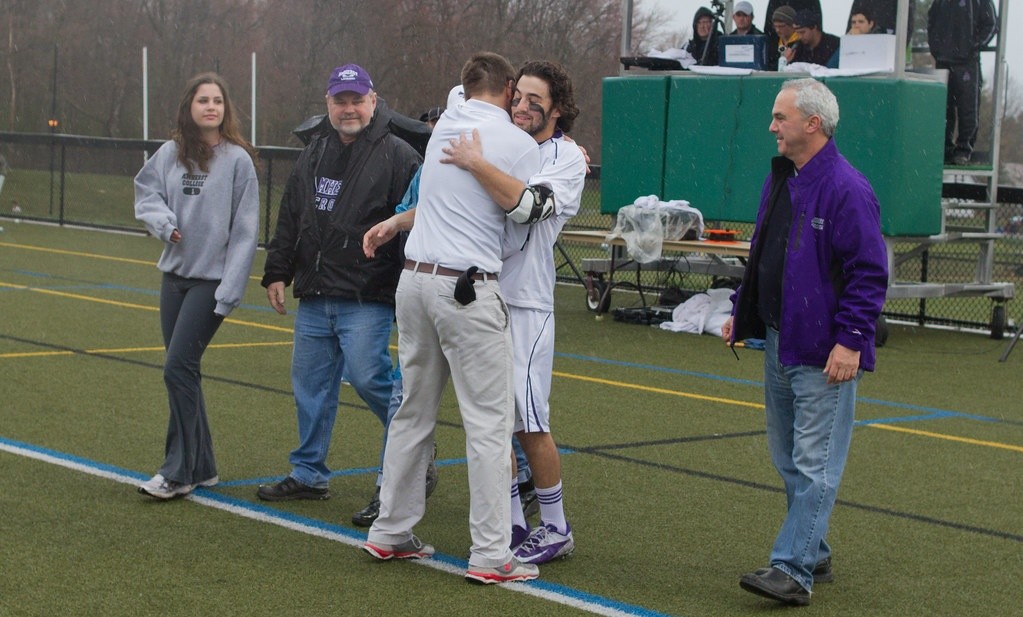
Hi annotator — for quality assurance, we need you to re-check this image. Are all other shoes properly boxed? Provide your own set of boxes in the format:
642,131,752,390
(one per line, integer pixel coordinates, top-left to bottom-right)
945,151,954,165
954,148,975,165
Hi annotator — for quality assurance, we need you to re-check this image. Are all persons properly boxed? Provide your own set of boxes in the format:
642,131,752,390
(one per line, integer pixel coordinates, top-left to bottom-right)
729,2,762,35
351,85,539,527
134,72,263,499
362,51,592,585
721,78,889,606
363,61,587,566
767,5,797,71
825,9,887,68
783,8,840,66
926,0,999,164
686,7,724,66
257,64,439,500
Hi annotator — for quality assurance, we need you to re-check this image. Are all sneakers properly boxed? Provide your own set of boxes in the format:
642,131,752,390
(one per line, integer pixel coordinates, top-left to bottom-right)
256,475,331,500
513,522,574,563
363,539,436,561
191,472,219,489
352,486,382,527
424,442,441,499
466,553,540,585
519,479,540,517
140,473,198,498
509,524,533,550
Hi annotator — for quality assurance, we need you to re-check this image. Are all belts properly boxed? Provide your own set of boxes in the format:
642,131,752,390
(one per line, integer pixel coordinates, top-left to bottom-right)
403,259,498,280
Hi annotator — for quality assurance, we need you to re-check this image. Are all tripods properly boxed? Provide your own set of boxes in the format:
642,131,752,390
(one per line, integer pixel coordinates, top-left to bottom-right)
699,0,728,66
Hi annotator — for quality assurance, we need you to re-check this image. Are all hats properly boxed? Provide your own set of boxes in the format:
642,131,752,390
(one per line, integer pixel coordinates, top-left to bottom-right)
791,8,820,31
731,1,753,17
326,63,373,98
772,6,797,26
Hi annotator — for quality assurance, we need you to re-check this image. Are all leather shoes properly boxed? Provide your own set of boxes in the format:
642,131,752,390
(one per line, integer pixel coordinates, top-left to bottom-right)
741,564,811,606
756,558,833,584
